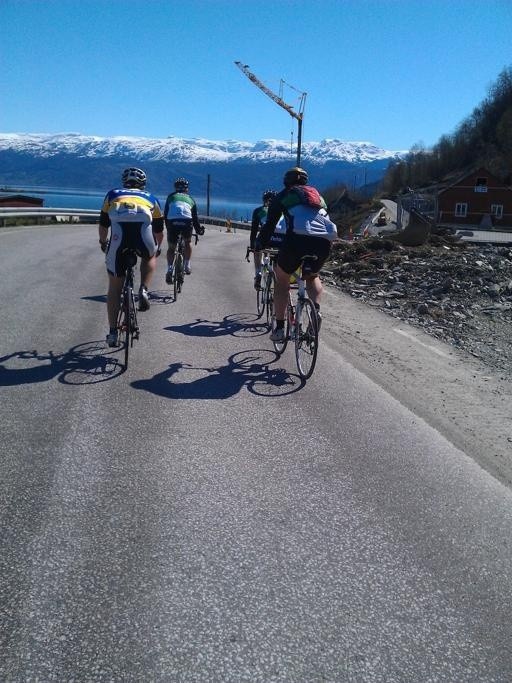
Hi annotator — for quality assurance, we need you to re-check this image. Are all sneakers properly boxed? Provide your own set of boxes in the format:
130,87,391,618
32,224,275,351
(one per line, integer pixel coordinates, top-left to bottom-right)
269,327,285,341
105,332,118,348
306,309,323,337
138,284,151,311
253,271,262,290
165,271,174,285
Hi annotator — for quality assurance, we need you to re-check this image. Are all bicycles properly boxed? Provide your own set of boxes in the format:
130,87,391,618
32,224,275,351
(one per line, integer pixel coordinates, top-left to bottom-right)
167,227,204,301
244,245,319,380
104,252,147,370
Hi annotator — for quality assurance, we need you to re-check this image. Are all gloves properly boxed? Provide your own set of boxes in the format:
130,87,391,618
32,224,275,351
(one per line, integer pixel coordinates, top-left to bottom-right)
98,239,111,254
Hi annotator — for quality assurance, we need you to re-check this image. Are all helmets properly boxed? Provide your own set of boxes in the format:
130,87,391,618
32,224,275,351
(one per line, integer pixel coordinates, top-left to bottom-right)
282,166,308,186
121,166,147,191
262,189,281,206
174,176,189,193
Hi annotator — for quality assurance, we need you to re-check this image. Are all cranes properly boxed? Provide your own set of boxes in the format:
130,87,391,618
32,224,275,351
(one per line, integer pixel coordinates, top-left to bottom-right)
233,60,309,167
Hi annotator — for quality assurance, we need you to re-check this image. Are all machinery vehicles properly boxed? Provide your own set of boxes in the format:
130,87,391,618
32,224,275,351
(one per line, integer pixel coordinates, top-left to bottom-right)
378,212,387,225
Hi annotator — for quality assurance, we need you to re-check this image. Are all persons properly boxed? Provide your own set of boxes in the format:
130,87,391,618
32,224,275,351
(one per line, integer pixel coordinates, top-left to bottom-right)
249,188,287,290
255,165,335,342
162,177,206,284
98,164,164,346
